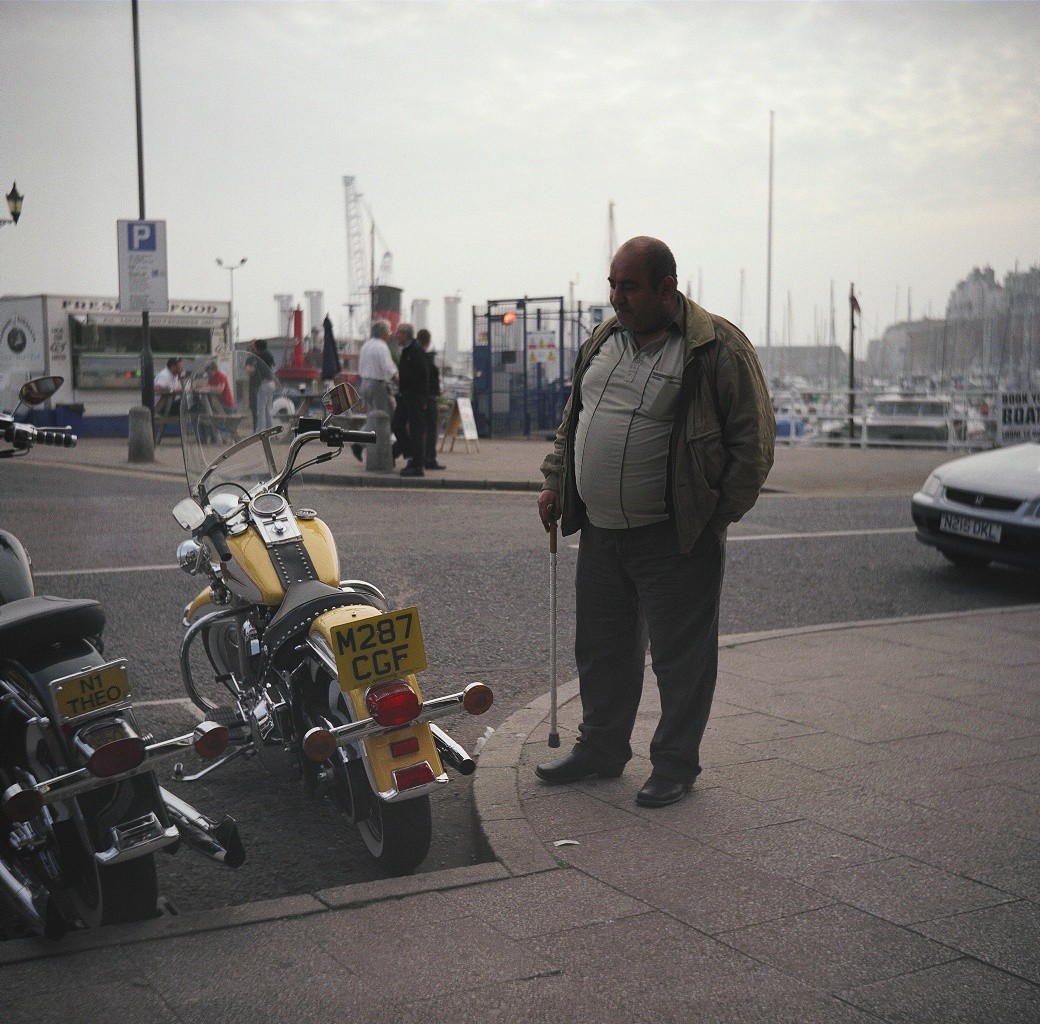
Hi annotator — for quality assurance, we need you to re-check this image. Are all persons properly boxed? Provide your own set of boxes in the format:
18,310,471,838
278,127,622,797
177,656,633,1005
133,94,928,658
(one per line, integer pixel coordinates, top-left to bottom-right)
351,319,447,476
246,338,272,434
538,236,776,805
772,407,818,436
152,355,232,417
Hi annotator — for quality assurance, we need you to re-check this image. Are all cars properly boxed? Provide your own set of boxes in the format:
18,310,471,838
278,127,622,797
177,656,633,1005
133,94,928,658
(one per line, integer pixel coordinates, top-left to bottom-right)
910,436,1040,574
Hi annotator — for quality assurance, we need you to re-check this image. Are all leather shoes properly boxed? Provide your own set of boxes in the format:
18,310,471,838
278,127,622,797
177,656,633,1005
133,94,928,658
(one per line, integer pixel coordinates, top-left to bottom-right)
635,772,696,805
535,748,627,782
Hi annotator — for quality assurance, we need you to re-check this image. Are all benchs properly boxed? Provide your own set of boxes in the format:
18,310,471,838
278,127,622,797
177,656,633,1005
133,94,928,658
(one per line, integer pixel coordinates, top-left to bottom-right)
152,411,243,428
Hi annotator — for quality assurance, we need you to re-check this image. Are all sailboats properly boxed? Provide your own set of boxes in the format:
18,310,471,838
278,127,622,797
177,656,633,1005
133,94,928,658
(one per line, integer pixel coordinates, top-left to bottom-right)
722,110,1035,448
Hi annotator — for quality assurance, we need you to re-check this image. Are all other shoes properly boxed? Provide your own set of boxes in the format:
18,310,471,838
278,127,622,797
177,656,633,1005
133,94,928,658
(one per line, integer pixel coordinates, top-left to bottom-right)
426,461,447,471
399,466,426,478
351,444,363,462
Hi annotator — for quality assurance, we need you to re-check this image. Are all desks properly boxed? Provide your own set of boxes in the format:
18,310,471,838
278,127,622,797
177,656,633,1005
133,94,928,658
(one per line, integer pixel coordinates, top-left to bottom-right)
155,383,224,446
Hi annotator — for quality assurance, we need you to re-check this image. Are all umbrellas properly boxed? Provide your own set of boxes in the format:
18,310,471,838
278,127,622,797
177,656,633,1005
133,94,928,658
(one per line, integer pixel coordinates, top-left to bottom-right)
321,318,341,391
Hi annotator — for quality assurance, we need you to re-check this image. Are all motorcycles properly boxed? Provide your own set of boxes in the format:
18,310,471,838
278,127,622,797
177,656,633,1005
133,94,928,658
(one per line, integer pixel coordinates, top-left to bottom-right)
0,376,252,943
165,381,495,877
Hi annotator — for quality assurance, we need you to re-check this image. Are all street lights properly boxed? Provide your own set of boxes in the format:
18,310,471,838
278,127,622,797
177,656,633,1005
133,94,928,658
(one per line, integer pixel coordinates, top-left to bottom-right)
215,257,250,380
